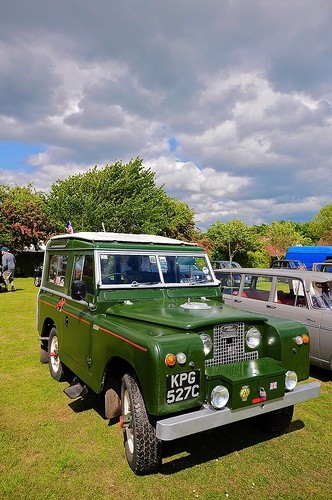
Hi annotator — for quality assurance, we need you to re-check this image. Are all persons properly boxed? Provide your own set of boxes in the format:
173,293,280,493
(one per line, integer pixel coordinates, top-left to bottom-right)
0,247,16,290
292,265,315,298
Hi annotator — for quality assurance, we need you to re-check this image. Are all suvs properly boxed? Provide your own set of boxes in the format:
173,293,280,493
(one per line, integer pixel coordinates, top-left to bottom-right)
36,232,321,477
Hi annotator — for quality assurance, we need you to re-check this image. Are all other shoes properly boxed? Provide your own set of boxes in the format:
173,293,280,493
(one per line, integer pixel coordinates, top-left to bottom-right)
11,286,14,291
3,288,8,292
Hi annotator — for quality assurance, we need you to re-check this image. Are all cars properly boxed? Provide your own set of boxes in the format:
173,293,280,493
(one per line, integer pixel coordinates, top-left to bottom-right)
262,259,302,282
209,260,244,288
34,256,71,288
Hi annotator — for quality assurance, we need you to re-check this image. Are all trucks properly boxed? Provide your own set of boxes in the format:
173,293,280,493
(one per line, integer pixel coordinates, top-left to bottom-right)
286,245,332,274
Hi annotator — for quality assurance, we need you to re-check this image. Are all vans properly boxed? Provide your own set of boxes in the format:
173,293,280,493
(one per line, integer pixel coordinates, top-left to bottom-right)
209,268,332,375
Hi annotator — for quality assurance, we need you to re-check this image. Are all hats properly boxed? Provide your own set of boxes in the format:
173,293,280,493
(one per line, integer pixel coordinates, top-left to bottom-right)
0,247,7,251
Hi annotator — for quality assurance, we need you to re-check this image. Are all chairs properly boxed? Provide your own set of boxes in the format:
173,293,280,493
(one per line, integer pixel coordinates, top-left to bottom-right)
241,289,259,299
123,269,143,283
276,290,295,304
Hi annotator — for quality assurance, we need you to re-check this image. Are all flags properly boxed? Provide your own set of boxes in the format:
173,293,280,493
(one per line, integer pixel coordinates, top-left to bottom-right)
65,221,74,234
